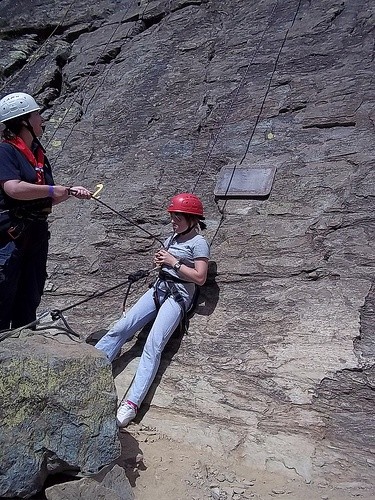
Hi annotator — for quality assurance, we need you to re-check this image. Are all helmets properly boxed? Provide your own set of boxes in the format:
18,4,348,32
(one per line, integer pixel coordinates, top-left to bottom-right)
168,194,203,215
0,92,41,123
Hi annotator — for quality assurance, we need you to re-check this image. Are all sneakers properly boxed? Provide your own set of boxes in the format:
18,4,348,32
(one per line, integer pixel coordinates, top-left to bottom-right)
116,402,136,428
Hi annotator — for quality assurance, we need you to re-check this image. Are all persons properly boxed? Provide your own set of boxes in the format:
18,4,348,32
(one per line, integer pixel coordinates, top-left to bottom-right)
94,192,210,428
0,92,94,332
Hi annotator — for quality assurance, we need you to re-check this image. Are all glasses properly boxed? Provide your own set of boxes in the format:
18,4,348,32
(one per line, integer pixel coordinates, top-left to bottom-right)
170,214,185,221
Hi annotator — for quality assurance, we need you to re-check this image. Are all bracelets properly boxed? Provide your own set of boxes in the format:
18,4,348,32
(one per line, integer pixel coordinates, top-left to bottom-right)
173,261,183,272
48,185,54,198
